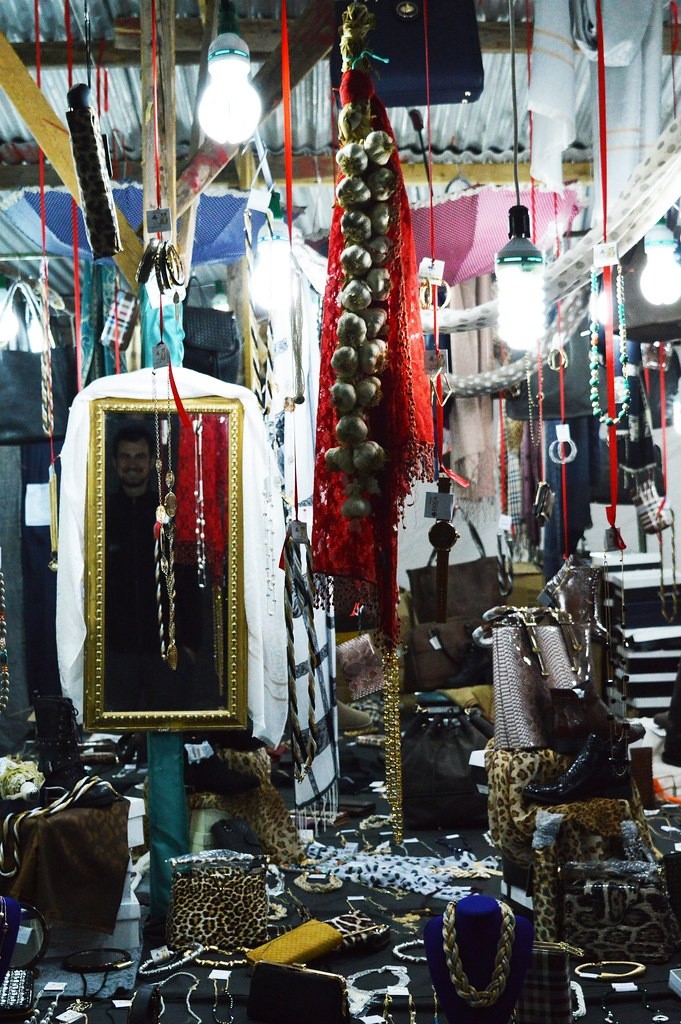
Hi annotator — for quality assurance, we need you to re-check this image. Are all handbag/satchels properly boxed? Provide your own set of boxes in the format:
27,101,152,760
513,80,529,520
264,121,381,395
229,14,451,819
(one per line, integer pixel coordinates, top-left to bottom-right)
0,968,34,1021
164,817,269,950
247,227,681,1024
127,984,160,1024
328,0,484,109
0,895,21,981
178,277,239,383
0,280,68,446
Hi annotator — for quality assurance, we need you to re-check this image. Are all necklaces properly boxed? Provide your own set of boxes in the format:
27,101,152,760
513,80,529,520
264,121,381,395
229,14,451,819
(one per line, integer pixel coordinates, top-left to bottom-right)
279,532,322,779
193,412,210,592
149,339,187,679
499,310,677,632
0,775,673,1024
442,897,517,1009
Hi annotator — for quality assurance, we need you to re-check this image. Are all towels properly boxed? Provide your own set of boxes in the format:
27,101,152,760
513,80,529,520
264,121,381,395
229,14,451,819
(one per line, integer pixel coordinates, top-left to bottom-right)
1,796,132,937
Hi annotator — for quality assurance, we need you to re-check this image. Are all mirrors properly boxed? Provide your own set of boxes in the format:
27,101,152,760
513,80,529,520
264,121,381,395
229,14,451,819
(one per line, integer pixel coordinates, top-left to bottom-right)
84,399,247,730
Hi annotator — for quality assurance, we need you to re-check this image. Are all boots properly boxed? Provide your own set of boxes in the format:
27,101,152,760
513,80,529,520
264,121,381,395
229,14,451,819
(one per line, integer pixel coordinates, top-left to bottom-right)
32,694,115,805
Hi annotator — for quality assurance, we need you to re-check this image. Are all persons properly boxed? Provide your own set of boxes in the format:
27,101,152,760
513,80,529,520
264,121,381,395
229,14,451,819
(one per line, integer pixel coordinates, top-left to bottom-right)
106,424,178,711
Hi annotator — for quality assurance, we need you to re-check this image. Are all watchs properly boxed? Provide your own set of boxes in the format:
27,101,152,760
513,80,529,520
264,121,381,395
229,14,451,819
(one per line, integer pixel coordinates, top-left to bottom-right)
427,434,461,627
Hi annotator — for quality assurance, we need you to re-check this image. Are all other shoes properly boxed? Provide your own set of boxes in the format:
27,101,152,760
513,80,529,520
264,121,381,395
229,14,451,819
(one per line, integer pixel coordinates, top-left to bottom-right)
183,741,261,794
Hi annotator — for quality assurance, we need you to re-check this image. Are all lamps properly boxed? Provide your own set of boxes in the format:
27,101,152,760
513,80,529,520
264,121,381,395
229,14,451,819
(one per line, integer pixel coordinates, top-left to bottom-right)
196,1,264,146
493,1,550,352
640,225,681,308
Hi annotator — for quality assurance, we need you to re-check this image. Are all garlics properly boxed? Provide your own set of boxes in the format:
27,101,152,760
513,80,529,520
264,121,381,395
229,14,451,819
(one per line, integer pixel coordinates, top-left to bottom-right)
324,131,395,520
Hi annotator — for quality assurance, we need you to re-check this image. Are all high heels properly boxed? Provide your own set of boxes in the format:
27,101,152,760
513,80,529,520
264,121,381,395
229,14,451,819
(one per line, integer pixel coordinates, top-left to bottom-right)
88,731,147,780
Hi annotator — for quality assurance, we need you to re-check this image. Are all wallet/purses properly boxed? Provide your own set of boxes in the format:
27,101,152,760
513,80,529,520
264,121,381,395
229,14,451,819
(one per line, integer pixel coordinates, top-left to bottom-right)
99,265,139,350
